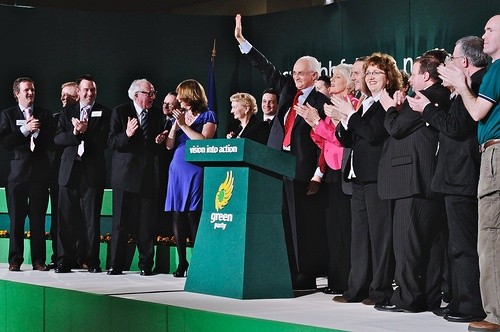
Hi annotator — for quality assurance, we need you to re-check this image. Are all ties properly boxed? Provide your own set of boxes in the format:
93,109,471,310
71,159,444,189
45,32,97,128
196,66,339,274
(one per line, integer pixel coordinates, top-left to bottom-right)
139,109,147,133
282,89,303,147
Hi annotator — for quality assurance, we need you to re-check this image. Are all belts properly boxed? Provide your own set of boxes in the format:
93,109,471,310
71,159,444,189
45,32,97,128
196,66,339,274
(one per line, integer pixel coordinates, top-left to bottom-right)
478,138,500,152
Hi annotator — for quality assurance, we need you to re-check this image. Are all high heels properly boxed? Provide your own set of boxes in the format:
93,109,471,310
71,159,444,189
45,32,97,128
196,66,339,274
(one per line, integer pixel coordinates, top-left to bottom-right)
172,261,190,278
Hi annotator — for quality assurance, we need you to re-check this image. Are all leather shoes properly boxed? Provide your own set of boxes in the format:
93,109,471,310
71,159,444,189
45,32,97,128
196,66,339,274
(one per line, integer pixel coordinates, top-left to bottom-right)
373,302,420,312
466,319,500,331
432,305,484,322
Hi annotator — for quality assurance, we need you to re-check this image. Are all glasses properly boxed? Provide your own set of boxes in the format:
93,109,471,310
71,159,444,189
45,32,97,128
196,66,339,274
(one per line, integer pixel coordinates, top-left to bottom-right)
138,89,158,98
451,55,466,63
364,69,386,77
162,100,175,107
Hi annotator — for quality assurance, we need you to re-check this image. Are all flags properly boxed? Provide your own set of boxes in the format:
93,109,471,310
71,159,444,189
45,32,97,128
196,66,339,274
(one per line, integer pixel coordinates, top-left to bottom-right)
207,58,215,138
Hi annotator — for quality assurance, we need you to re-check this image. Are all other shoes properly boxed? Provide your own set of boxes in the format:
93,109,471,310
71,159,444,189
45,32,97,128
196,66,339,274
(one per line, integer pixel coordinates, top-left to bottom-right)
140,267,151,276
364,295,384,304
9,264,21,271
332,294,368,302
55,265,71,273
33,263,50,272
87,263,102,274
320,282,346,292
107,267,122,275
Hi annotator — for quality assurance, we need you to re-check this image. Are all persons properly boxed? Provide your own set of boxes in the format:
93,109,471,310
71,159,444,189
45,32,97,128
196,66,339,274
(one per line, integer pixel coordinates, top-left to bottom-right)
162,79,218,277
234,14,333,291
225,92,267,143
294,14,500,332
261,90,278,125
54,77,112,273
0,78,57,271
151,90,184,274
48,82,88,268
108,79,167,276
